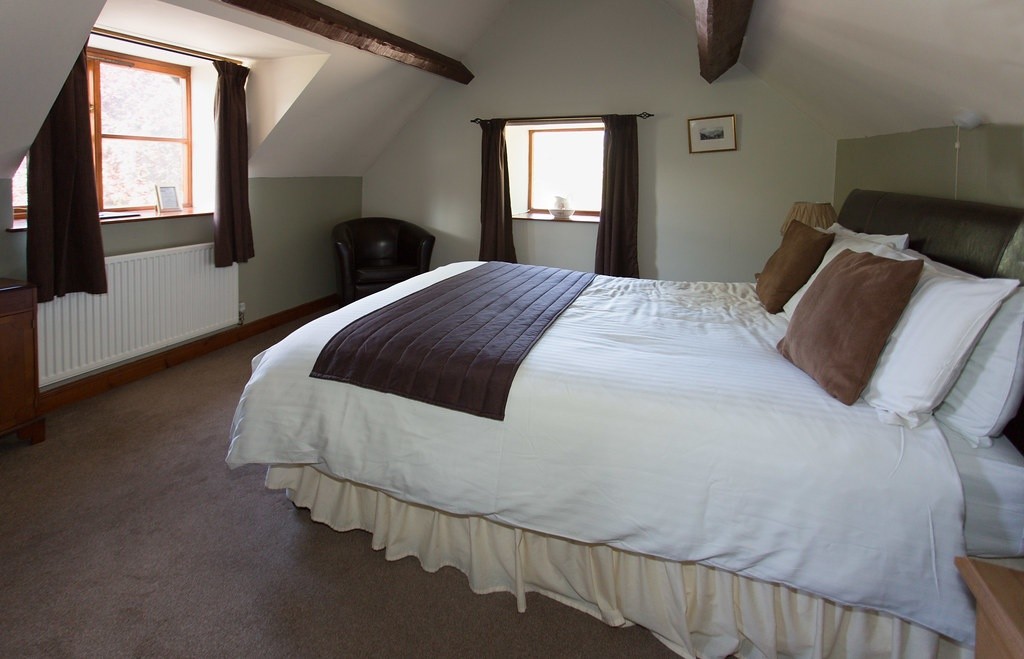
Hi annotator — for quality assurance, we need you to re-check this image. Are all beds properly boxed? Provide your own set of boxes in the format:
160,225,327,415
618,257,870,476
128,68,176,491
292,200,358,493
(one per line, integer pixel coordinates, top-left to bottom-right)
224,123,1024,659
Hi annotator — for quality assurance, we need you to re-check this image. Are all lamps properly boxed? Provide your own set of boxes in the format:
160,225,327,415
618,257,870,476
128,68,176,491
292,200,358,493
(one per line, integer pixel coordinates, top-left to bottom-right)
779,202,838,233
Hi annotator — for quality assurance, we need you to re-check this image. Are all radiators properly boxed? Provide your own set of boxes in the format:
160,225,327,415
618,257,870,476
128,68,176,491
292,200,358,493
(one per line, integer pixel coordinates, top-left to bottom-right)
38,243,240,394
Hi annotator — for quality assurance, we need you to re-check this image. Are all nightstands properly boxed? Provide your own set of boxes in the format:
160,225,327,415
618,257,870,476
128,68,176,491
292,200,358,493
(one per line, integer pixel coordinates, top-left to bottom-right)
953,557,1024,659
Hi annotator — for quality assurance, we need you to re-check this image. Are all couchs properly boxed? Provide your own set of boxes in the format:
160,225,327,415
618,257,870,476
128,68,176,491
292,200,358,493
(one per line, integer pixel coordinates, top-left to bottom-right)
332,217,436,308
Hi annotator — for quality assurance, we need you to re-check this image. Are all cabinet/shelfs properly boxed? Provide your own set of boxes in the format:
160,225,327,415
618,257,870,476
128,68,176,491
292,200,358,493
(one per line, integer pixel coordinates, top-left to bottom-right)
0,278,45,447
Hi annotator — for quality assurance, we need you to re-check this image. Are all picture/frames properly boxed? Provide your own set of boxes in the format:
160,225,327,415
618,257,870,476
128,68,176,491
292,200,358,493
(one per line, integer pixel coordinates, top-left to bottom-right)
687,113,737,154
155,185,182,213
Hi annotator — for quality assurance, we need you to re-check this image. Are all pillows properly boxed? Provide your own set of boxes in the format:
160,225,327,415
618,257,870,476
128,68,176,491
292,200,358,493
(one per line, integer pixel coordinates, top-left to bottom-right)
860,245,1020,429
826,223,910,259
782,227,896,318
776,249,925,407
905,250,1024,447
756,219,834,314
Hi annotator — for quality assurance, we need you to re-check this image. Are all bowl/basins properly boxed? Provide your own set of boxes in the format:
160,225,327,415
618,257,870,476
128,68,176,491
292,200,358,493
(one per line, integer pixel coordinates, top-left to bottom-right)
549,210,575,219
